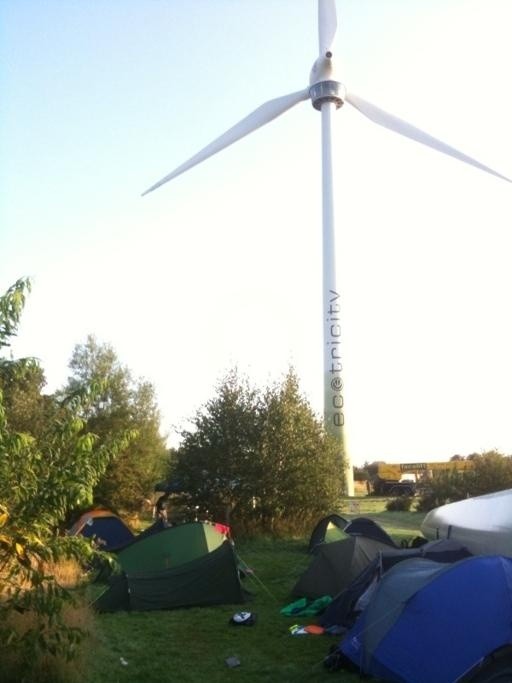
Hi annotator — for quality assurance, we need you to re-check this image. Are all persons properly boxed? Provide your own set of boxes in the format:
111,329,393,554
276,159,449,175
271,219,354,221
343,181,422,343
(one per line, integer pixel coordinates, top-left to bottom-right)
155,491,172,526
